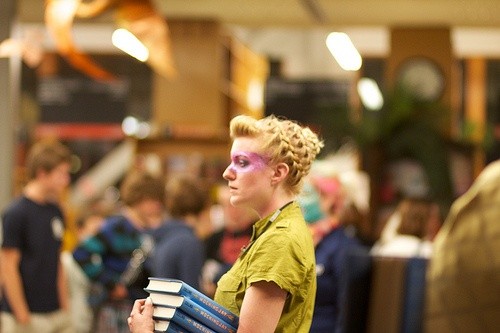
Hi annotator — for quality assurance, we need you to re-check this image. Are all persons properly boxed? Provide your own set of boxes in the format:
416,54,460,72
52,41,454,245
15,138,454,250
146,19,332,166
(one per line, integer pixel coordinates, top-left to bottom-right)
128,115,323,333
73,177,166,332
296,160,371,333
150,176,210,291
1,141,69,333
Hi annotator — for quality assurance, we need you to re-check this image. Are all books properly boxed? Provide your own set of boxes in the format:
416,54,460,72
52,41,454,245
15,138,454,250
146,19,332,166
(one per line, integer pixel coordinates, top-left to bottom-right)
143,278,240,333
